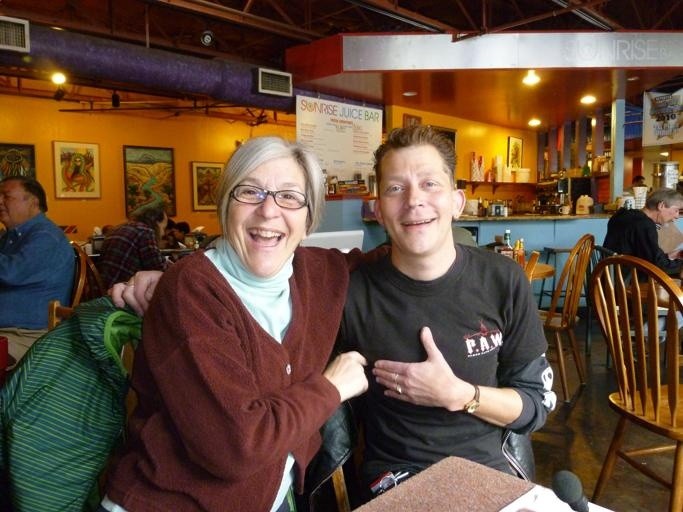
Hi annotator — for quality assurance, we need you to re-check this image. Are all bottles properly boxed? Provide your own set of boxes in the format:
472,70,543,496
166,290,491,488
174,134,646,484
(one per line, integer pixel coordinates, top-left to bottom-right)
513,239,519,260
478,197,483,216
504,205,508,217
518,238,526,269
583,159,590,176
498,230,514,260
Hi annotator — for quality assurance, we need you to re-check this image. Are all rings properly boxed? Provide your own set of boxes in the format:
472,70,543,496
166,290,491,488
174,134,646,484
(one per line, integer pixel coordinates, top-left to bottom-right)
396,384,401,394
125,284,134,288
394,374,397,382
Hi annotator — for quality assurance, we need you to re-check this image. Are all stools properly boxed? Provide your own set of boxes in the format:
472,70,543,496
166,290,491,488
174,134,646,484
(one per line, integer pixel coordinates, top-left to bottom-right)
539,246,597,318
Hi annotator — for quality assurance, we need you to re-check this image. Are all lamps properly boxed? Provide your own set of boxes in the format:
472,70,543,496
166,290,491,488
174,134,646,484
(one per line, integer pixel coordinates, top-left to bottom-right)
200,29,214,45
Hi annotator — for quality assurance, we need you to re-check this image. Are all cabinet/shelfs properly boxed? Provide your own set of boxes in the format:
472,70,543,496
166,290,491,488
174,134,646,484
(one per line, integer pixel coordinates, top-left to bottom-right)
458,178,555,191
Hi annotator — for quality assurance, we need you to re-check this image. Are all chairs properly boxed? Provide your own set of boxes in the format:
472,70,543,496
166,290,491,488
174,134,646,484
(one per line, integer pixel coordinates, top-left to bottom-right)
585,244,636,368
537,234,595,403
67,241,86,308
587,253,683,512
87,256,106,300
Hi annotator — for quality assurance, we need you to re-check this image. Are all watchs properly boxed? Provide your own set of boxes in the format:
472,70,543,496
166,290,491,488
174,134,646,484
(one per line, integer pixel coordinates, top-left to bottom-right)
465,384,480,415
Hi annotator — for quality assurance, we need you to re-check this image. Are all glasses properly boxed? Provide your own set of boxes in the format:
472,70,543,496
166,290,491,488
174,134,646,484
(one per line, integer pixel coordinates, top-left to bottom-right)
230,184,308,210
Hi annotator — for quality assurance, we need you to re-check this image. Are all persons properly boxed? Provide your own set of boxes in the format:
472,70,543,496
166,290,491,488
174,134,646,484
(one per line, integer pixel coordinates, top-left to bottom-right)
678,181,683,194
163,218,190,260
97,137,368,512
102,224,112,234
626,175,645,195
97,206,174,295
109,124,557,506
0,175,79,375
603,188,683,363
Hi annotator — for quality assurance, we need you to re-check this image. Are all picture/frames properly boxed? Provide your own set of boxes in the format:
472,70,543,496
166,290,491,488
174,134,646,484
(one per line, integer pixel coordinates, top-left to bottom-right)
52,140,102,199
0,143,36,180
123,143,177,218
191,161,227,211
507,136,523,168
